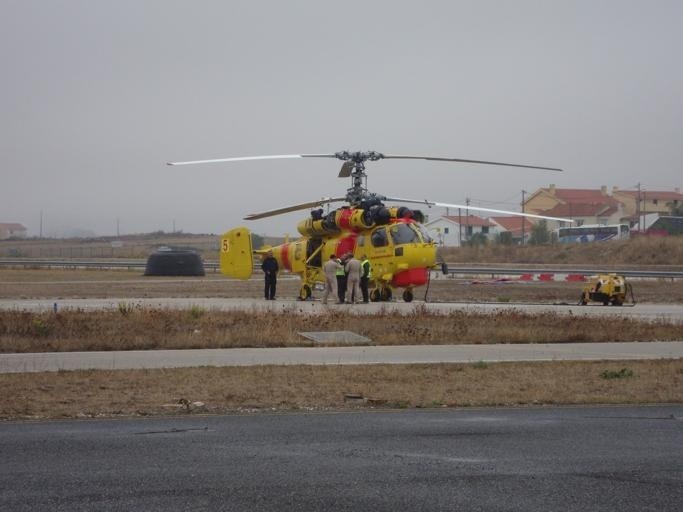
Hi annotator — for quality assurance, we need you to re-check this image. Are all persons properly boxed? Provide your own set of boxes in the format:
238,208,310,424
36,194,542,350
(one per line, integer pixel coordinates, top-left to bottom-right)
336,252,347,302
358,253,372,303
260,251,279,300
343,253,361,304
320,253,342,305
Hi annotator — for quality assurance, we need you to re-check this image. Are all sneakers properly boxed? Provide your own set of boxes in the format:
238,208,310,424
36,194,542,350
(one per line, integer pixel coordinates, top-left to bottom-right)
335,298,368,304
265,297,275,300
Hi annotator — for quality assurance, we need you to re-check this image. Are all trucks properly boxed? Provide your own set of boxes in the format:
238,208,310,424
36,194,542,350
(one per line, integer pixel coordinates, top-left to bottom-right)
582,274,627,305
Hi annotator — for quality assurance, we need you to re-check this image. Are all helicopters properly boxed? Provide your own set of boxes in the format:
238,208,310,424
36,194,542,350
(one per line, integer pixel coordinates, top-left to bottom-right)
166,153,575,302
